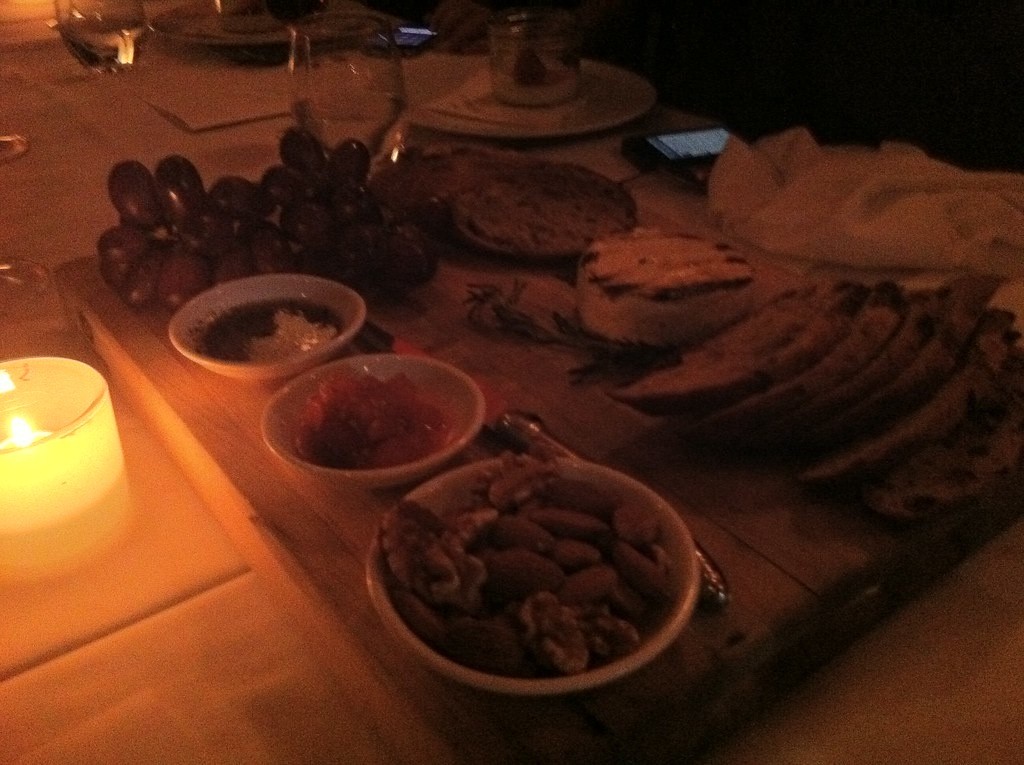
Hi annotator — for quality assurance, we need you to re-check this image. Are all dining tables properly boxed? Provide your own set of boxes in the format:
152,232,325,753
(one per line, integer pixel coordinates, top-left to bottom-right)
0,0,1023,765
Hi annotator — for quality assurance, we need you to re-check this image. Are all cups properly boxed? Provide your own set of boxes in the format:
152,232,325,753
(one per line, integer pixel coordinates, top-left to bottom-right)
286,12,406,156
487,13,578,108
53,0,146,73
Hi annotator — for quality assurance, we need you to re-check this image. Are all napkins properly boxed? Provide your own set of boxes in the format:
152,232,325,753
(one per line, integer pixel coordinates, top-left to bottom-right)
426,64,603,125
711,126,1024,278
134,60,292,132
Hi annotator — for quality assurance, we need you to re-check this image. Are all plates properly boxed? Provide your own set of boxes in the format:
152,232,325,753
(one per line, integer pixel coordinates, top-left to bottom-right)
146,0,293,45
403,53,657,139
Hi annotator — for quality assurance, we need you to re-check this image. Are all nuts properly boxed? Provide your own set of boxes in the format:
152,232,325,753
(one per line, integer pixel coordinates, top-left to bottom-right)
379,454,677,677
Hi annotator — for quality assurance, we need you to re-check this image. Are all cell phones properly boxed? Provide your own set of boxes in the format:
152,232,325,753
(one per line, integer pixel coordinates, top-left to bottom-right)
624,126,730,173
363,26,440,59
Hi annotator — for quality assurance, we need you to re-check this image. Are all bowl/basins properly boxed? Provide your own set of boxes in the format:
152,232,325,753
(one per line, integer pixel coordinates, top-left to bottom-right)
365,455,702,697
168,272,369,383
261,353,486,489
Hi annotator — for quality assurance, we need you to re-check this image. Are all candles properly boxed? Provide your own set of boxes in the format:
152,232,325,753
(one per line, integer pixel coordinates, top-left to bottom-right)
0,357,134,574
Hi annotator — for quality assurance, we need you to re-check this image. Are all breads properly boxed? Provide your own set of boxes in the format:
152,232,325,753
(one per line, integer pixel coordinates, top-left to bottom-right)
605,269,1024,523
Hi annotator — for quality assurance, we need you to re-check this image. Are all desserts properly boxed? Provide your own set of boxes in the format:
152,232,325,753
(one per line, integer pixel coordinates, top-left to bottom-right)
576,225,751,348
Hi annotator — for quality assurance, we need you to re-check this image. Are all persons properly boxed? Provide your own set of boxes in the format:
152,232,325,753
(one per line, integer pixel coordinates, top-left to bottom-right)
262,0,635,45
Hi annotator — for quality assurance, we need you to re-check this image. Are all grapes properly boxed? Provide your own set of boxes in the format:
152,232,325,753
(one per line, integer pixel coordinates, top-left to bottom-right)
98,127,438,314
